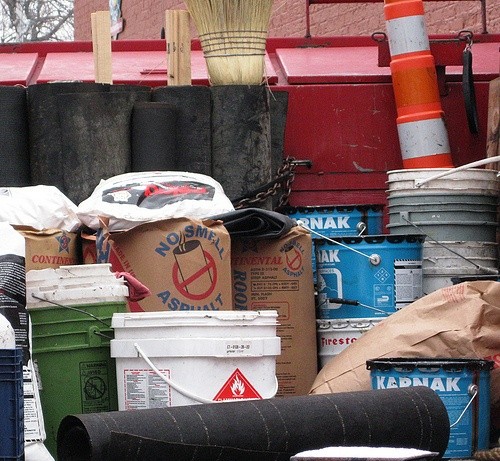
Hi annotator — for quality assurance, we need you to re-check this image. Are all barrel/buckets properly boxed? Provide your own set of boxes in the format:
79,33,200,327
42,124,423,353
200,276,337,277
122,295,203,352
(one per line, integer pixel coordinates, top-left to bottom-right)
282,203,386,287
26,263,281,460
317,297,390,371
385,156,499,297
311,233,427,321
366,357,494,461
